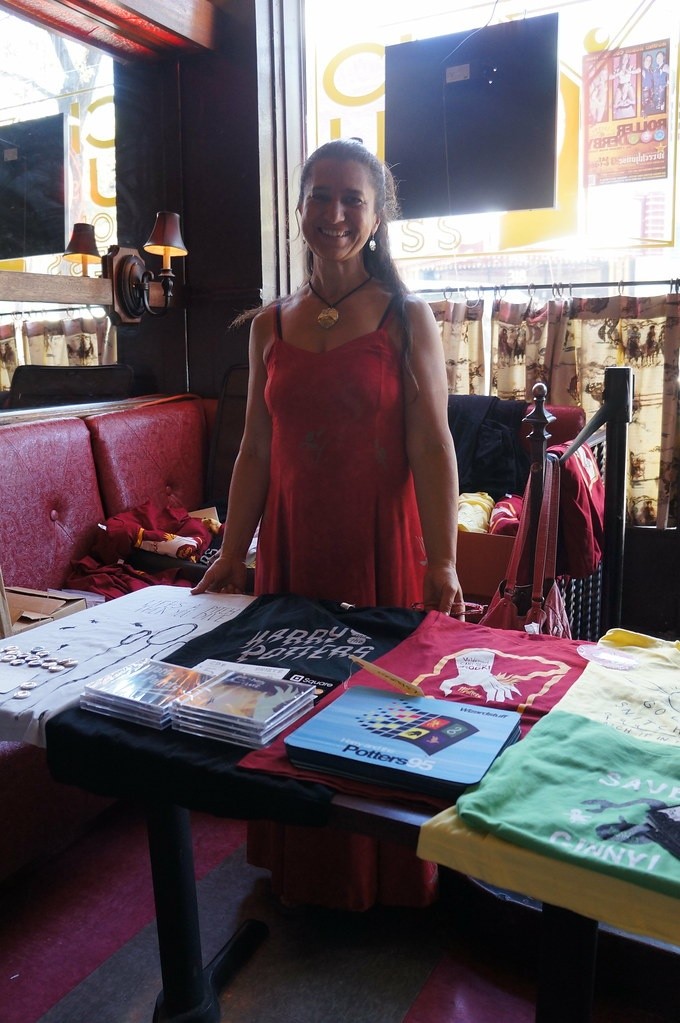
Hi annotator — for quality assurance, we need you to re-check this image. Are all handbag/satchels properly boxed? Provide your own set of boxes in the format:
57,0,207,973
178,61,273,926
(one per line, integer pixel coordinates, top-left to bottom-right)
477,452,574,639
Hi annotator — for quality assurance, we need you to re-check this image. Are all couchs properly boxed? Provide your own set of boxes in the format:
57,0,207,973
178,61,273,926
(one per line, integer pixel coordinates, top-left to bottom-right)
0,397,588,858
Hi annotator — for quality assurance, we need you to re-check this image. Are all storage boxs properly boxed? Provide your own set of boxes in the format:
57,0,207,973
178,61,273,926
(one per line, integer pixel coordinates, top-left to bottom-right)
456,531,516,600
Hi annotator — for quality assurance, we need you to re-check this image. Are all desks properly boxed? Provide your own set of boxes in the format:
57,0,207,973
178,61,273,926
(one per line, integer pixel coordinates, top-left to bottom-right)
0,581,680,1023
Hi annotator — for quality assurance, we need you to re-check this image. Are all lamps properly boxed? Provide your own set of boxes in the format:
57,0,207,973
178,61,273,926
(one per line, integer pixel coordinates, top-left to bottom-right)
61,223,102,277
108,210,190,324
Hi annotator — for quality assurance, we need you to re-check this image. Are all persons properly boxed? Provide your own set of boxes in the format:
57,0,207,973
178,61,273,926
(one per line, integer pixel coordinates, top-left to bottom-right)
188,137,467,934
587,50,670,122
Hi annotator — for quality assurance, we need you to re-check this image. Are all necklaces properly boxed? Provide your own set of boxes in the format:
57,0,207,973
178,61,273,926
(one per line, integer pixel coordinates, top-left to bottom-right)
307,271,373,330
